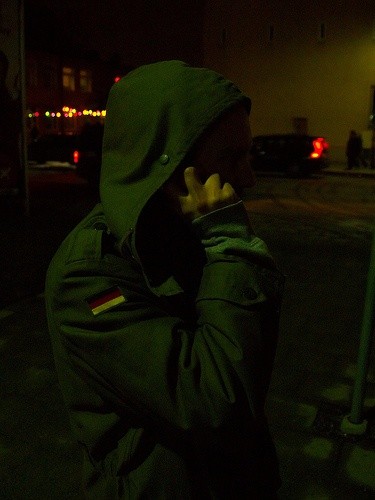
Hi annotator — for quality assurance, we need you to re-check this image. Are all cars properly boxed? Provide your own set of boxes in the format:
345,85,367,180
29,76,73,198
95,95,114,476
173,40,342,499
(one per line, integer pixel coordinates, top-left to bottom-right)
27,133,77,165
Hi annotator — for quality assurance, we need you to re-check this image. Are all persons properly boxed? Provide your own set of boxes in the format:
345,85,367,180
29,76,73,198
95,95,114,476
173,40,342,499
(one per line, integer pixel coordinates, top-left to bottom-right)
38,59,295,500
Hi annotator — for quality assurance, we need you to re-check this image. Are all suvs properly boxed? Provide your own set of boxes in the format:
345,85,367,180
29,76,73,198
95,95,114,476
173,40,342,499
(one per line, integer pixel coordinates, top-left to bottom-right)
249,132,329,178
